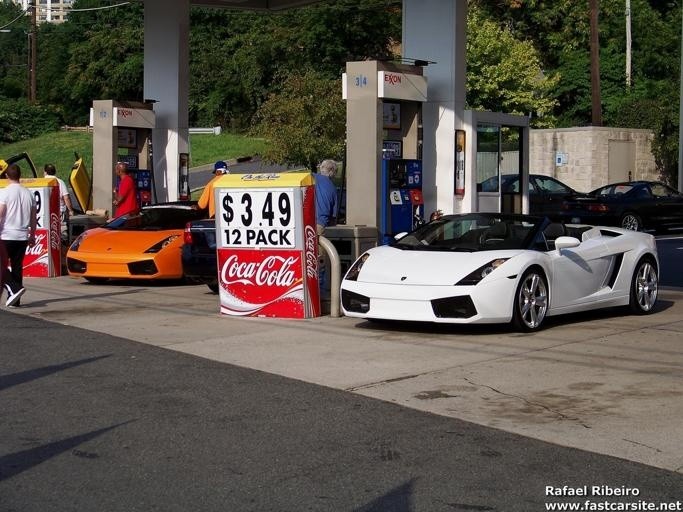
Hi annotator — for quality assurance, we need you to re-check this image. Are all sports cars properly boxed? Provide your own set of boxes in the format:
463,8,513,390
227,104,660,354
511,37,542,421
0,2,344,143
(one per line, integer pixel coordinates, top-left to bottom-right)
66,201,208,286
340,212,660,334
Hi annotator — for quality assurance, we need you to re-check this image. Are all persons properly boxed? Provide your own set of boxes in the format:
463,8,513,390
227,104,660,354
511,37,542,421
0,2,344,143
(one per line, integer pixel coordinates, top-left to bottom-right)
0,163,38,311
107,159,138,225
43,160,75,225
309,157,343,233
189,160,231,221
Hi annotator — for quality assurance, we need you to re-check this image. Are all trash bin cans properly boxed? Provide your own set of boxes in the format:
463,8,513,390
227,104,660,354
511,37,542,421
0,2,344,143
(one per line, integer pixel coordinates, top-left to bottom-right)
324,224,378,316
69,214,105,247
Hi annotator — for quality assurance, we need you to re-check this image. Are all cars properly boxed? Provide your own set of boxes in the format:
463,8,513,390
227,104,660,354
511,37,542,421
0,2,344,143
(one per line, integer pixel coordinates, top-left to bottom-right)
477,174,683,232
181,213,218,294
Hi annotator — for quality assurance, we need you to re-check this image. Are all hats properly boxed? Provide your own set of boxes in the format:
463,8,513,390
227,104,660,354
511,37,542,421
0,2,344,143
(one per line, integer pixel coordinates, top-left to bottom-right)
212,160,227,174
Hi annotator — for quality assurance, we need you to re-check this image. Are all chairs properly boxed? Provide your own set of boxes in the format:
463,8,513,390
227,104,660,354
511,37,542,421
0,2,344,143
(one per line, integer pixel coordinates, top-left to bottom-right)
478,222,506,250
616,191,622,195
641,190,652,197
534,223,567,252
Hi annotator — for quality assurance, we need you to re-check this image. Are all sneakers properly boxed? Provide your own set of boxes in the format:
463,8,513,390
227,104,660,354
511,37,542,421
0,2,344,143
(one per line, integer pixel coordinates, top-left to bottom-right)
5,287,26,308
